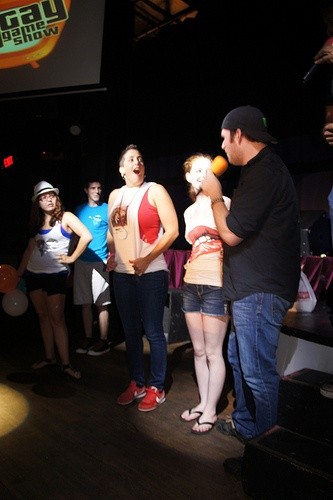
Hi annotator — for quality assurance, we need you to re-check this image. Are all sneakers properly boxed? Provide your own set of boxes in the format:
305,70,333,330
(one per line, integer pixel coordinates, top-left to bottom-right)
137,386,165,411
115,380,146,404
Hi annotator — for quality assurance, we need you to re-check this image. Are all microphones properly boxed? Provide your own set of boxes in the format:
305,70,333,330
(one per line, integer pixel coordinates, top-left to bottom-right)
194,154,228,197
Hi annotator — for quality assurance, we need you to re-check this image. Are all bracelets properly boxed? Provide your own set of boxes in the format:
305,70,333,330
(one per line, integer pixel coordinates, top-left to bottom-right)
107,253,115,260
211,198,224,209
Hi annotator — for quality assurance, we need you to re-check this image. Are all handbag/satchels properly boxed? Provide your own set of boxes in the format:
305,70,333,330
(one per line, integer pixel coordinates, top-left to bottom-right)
288,270,317,313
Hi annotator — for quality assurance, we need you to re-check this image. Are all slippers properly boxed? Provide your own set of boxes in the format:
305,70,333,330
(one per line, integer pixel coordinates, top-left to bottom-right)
191,417,216,435
179,407,203,422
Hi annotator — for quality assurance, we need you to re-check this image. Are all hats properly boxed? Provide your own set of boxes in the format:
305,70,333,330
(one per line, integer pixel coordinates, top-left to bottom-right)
32,180,60,204
220,106,278,145
39,194,57,201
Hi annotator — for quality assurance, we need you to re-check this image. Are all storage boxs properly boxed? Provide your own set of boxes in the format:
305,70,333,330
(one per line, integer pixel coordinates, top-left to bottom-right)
278,368,333,443
240,427,333,500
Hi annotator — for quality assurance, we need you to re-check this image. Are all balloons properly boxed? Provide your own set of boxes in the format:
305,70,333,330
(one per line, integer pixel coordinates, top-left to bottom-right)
3,289,28,316
0,265,20,293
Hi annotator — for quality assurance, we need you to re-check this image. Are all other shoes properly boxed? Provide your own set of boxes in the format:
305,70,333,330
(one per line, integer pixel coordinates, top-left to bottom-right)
214,418,234,436
76,337,94,353
63,364,80,379
88,339,110,355
31,357,56,369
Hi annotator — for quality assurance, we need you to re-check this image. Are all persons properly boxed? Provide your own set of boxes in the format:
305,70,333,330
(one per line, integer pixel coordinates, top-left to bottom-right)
314,35,333,401
181,153,231,434
200,107,300,480
105,145,180,412
16,181,93,379
73,176,110,356
